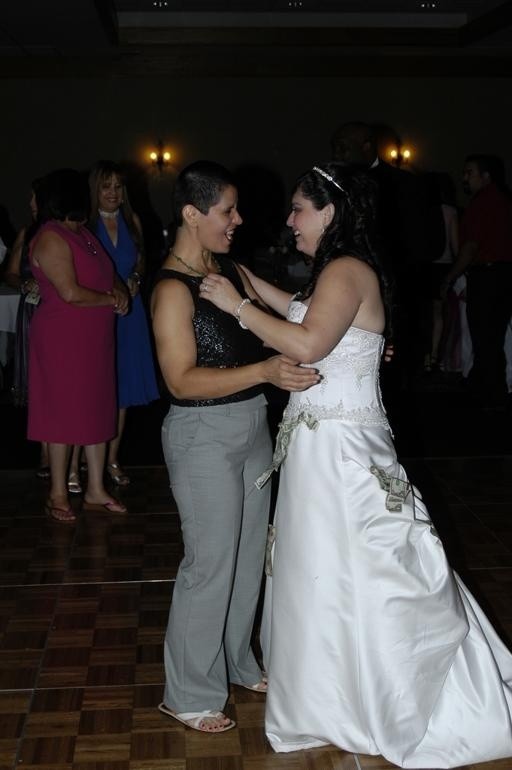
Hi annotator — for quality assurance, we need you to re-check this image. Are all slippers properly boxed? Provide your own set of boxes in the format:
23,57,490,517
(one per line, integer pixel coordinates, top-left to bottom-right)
39,459,130,523
159,701,236,733
245,676,271,693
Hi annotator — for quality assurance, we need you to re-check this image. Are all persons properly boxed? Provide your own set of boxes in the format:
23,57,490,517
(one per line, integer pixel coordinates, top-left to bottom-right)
145,156,398,734
196,158,512,769
419,153,512,415
3,159,161,524
332,121,446,461
